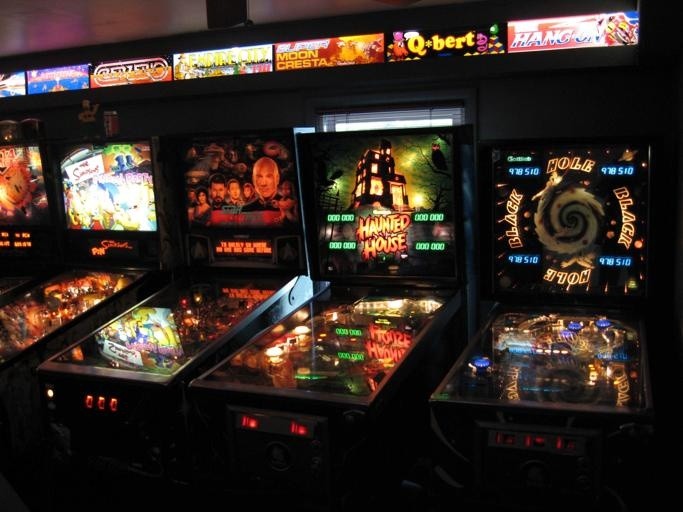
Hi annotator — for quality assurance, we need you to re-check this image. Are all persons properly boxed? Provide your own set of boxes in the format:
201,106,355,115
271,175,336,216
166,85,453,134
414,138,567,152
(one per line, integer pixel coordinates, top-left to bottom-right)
186,157,300,225
